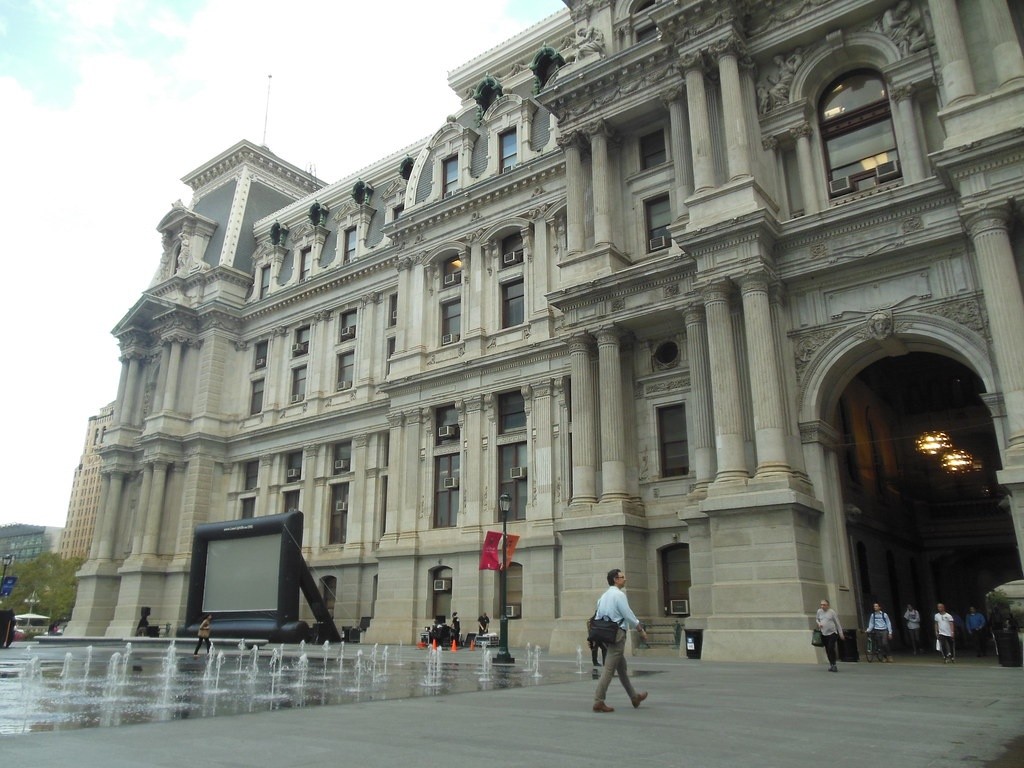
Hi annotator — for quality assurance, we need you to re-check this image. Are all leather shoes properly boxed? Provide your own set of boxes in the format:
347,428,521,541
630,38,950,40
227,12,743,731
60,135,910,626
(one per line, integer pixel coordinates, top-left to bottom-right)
593,702,614,712
631,691,648,708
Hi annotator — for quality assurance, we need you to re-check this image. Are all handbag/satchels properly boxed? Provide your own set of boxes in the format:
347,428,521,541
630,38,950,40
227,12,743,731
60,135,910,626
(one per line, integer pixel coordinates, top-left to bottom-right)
935,639,941,651
197,629,210,639
812,628,824,647
587,610,625,643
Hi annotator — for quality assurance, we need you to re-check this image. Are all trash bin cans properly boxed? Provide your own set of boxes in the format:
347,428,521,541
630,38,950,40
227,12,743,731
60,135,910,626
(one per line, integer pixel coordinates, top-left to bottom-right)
837,628,860,662
684,629,704,659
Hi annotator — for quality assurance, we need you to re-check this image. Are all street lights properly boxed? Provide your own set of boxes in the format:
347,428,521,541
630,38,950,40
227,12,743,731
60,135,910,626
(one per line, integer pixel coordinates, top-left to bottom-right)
0,553,13,599
491,490,515,664
24,590,41,626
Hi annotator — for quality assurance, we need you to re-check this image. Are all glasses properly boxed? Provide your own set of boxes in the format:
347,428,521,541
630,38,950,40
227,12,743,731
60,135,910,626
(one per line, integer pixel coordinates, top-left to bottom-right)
616,576,624,579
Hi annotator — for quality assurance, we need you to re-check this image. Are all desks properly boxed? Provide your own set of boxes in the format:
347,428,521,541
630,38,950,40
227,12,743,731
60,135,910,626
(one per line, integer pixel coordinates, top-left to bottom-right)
421,632,462,648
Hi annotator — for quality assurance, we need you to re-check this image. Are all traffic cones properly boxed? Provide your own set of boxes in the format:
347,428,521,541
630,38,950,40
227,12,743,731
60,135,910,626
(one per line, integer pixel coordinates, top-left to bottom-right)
432,639,437,650
469,640,476,651
450,640,457,651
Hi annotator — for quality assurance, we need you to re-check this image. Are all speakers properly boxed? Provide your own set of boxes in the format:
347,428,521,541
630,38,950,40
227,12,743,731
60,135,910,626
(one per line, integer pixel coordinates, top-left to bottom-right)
436,616,446,624
141,607,151,617
360,616,371,629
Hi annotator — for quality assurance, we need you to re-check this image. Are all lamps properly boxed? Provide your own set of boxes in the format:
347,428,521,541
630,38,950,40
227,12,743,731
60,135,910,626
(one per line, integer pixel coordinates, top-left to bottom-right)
915,375,983,478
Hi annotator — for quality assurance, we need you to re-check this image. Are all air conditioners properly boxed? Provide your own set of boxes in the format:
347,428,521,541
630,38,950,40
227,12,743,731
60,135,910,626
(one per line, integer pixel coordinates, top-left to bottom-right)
292,343,302,351
291,394,303,403
335,460,348,468
341,326,354,336
829,175,853,197
288,469,300,477
256,358,264,366
649,235,669,250
442,334,458,345
336,502,347,511
670,600,689,614
510,466,527,479
434,580,451,590
337,381,351,390
875,159,900,183
504,251,517,265
445,274,457,285
444,190,455,200
506,605,520,618
444,477,459,488
502,165,514,173
439,426,455,437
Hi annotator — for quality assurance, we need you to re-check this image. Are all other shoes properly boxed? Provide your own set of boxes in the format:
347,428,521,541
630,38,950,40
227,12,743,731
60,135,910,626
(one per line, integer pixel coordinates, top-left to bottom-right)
828,665,837,672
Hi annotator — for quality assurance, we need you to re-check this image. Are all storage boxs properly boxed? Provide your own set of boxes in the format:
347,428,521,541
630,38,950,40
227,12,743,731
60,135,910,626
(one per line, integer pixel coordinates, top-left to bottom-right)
475,635,499,647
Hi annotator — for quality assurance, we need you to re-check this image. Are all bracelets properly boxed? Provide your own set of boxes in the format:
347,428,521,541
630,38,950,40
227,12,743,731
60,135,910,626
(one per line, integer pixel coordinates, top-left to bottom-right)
638,628,644,632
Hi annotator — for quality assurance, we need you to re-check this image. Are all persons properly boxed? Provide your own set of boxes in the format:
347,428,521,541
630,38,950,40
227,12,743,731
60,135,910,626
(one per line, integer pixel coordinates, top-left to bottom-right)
935,604,1021,663
865,602,894,663
477,612,490,635
588,568,649,713
816,598,845,672
450,612,460,645
904,604,923,655
137,615,149,636
194,614,213,656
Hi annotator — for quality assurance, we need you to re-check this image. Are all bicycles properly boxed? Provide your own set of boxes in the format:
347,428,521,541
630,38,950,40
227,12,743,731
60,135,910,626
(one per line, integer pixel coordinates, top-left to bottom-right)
861,630,885,663
